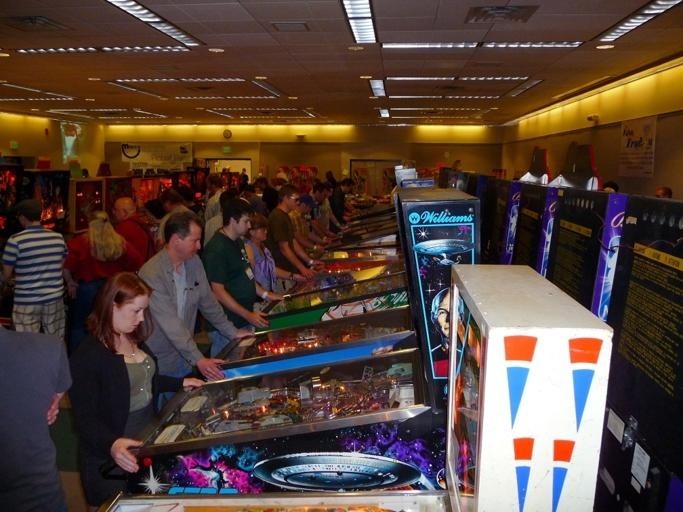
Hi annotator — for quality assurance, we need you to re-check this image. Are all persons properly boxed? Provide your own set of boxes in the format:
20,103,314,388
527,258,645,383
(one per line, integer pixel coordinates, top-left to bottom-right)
61,168,355,358
1,200,69,339
602,182,619,193
656,187,672,199
69,271,206,509
0,326,73,512
138,209,255,415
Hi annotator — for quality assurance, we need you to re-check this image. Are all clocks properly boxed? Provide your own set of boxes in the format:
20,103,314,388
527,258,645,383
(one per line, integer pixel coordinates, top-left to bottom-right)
223,129,232,138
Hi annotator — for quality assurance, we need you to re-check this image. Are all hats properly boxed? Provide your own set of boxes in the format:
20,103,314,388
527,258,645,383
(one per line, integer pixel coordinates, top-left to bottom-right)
301,194,318,208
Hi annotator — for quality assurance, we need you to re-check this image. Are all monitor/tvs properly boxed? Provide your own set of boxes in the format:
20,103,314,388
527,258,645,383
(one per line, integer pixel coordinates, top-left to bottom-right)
446,264,613,512
